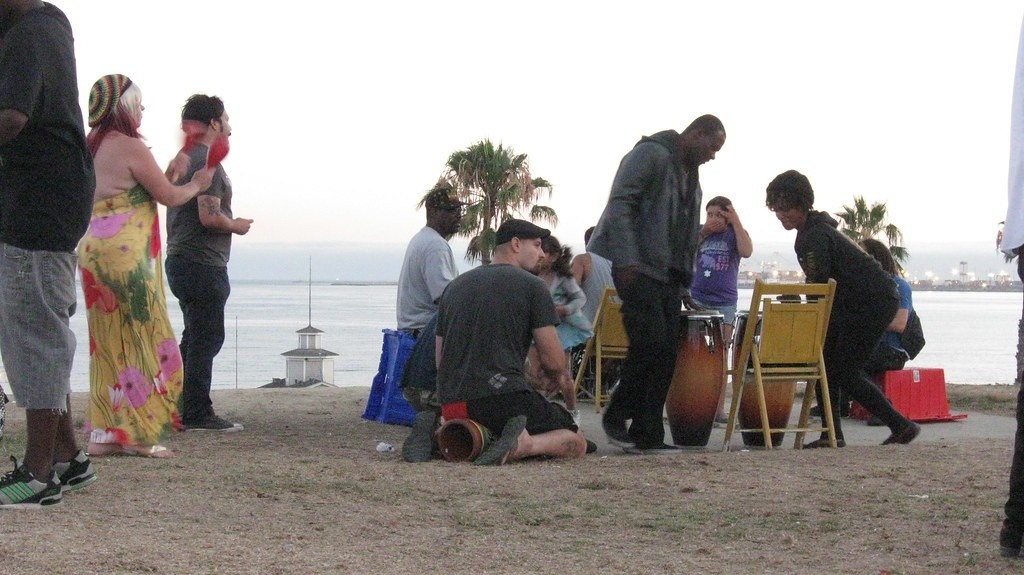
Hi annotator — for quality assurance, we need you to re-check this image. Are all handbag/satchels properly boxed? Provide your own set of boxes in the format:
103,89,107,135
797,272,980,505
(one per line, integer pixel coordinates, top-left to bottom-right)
363,328,417,425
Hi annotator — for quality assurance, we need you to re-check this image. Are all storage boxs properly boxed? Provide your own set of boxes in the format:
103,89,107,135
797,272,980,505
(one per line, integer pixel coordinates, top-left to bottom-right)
847,365,969,424
362,327,428,427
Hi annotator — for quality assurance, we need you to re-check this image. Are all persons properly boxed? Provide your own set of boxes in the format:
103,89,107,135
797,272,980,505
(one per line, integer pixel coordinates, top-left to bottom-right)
165,95,254,432
397,185,621,466
588,114,727,454
1000,15,1024,560
692,195,754,429
75,74,218,456
0,0,95,509
765,170,925,449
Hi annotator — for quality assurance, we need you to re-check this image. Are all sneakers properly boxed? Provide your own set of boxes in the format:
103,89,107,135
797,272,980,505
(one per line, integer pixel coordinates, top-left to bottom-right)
185,417,244,433
0,450,99,509
603,415,683,455
88,74,133,127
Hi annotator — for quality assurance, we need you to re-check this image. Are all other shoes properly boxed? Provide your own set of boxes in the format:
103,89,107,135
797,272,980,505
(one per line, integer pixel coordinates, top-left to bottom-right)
805,439,847,448
880,423,922,445
86,444,177,459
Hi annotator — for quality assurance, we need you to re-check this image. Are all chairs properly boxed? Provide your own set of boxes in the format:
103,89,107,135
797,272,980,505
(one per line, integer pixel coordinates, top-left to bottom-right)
571,287,627,413
718,278,837,449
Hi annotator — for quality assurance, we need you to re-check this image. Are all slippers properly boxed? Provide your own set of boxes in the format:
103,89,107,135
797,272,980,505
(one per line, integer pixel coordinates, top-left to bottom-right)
464,414,528,465
403,410,437,462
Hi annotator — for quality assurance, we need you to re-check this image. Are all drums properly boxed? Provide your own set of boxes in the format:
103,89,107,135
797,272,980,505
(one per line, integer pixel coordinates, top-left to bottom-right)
732,310,797,447
664,311,725,447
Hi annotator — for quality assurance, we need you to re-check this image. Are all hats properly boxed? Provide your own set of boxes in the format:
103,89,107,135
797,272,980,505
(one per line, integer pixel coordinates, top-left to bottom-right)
426,187,470,210
497,219,552,244
